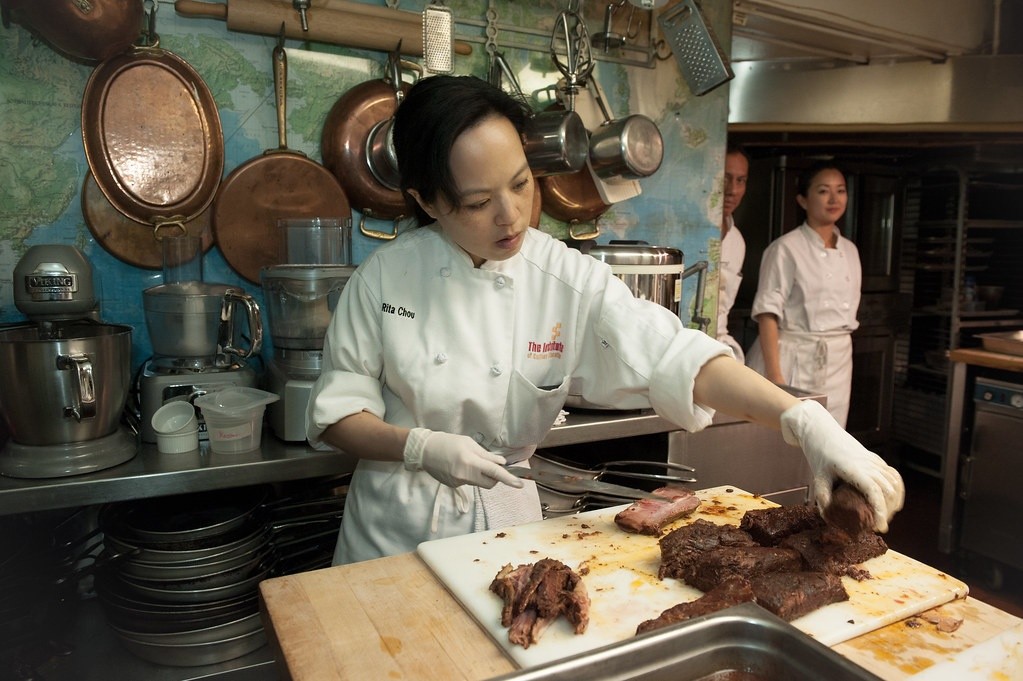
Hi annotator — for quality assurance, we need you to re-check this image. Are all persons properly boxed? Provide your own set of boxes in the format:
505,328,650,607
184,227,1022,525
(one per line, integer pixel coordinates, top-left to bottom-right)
743,159,862,430
304,73,904,568
716,145,753,365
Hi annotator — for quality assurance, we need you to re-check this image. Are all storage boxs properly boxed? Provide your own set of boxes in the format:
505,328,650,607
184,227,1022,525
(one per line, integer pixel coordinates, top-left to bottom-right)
195,389,281,457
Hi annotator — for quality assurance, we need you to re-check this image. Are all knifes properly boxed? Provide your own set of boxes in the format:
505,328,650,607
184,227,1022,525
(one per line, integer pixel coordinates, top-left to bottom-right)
500,464,672,504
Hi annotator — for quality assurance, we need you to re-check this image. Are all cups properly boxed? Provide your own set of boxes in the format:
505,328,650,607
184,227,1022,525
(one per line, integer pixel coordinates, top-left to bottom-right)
162,236,204,284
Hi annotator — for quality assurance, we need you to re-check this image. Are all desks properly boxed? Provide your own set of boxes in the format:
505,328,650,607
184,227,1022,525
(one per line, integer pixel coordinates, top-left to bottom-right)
939,347,1023,559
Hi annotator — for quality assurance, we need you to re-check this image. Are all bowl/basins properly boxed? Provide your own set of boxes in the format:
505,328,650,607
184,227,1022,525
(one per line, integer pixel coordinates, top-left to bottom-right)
199,405,267,456
150,400,200,455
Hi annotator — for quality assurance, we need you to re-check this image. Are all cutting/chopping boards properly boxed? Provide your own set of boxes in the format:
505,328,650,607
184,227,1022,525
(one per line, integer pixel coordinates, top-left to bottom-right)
415,483,971,673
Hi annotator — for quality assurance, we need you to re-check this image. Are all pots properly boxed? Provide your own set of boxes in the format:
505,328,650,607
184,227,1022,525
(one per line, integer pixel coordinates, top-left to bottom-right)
527,451,696,520
143,279,264,358
0,468,356,681
262,264,362,352
323,54,685,329
0,322,135,446
0,0,352,285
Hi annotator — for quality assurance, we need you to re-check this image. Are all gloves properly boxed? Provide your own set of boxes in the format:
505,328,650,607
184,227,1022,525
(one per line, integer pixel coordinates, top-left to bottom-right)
780,399,905,534
403,428,525,490
